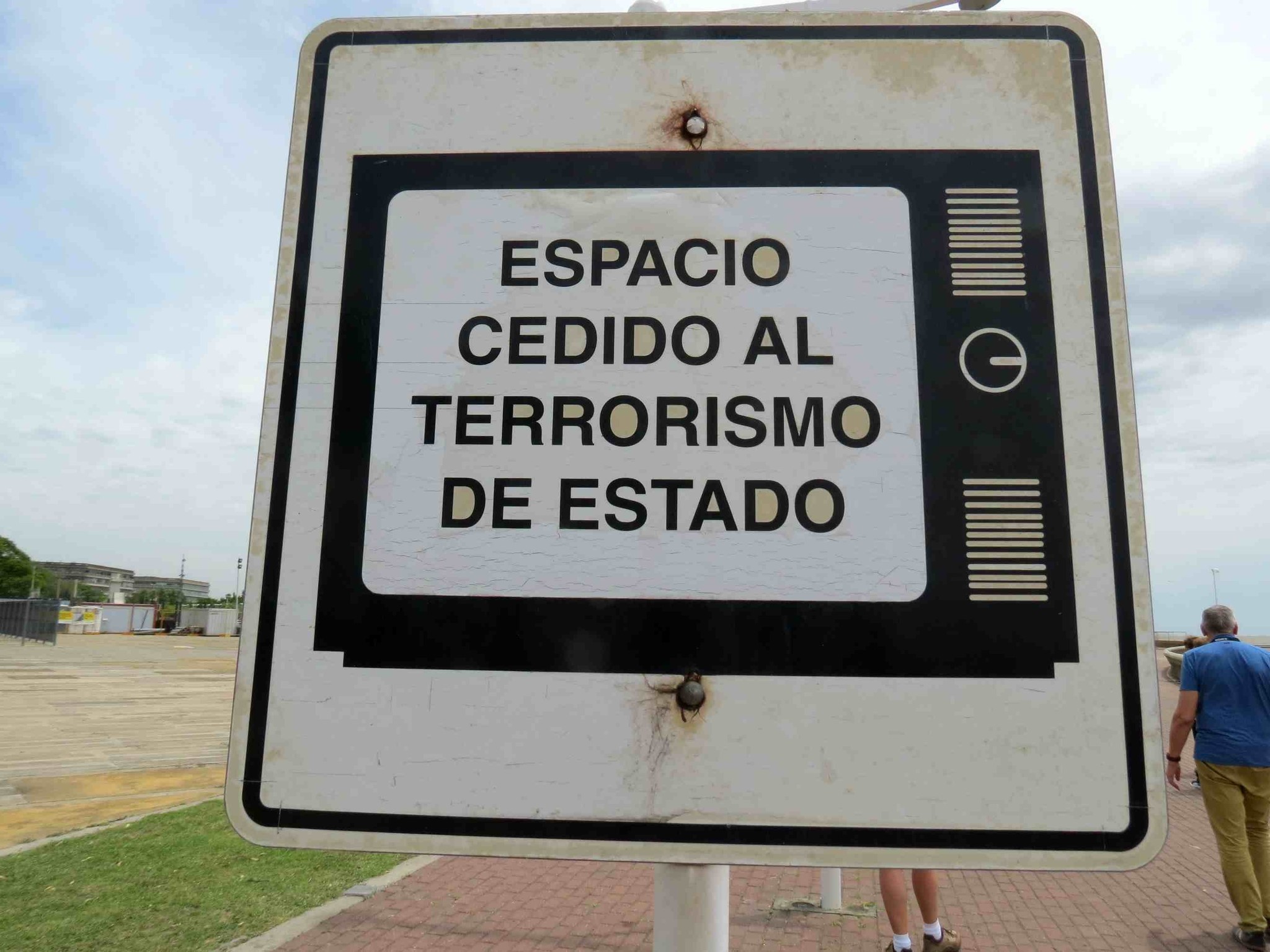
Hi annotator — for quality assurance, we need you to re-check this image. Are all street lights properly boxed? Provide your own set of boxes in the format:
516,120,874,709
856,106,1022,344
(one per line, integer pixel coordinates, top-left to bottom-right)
236,556,243,609
1211,568,1220,604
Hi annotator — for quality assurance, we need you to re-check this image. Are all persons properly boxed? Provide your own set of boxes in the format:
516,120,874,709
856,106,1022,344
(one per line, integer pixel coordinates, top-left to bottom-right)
1182,635,1215,791
876,868,963,952
1166,605,1270,951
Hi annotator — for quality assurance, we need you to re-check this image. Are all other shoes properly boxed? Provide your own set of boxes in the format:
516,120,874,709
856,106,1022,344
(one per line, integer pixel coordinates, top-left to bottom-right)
1190,781,1201,789
923,929,961,952
885,940,913,952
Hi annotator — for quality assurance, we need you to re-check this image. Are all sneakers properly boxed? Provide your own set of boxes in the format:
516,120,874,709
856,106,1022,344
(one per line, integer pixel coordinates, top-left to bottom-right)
1232,926,1266,951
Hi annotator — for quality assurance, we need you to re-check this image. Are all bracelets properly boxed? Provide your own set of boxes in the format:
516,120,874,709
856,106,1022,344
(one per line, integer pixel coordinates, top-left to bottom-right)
1167,752,1181,762
